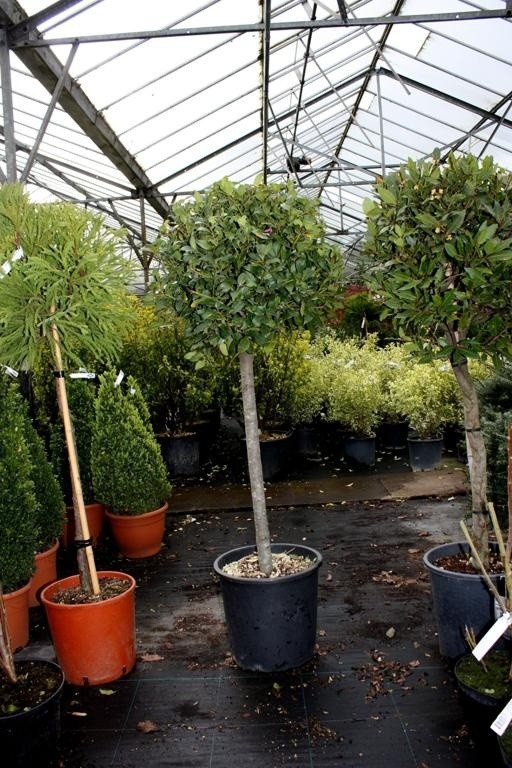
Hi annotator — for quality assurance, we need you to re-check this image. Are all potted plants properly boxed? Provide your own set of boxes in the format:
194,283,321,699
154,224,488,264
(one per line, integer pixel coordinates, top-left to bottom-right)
153,170,357,675
140,308,454,482
361,143,512,659
3,181,172,762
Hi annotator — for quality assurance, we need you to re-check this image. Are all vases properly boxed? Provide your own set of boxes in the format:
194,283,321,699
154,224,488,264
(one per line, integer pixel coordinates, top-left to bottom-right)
495,720,511,766
450,650,512,765
